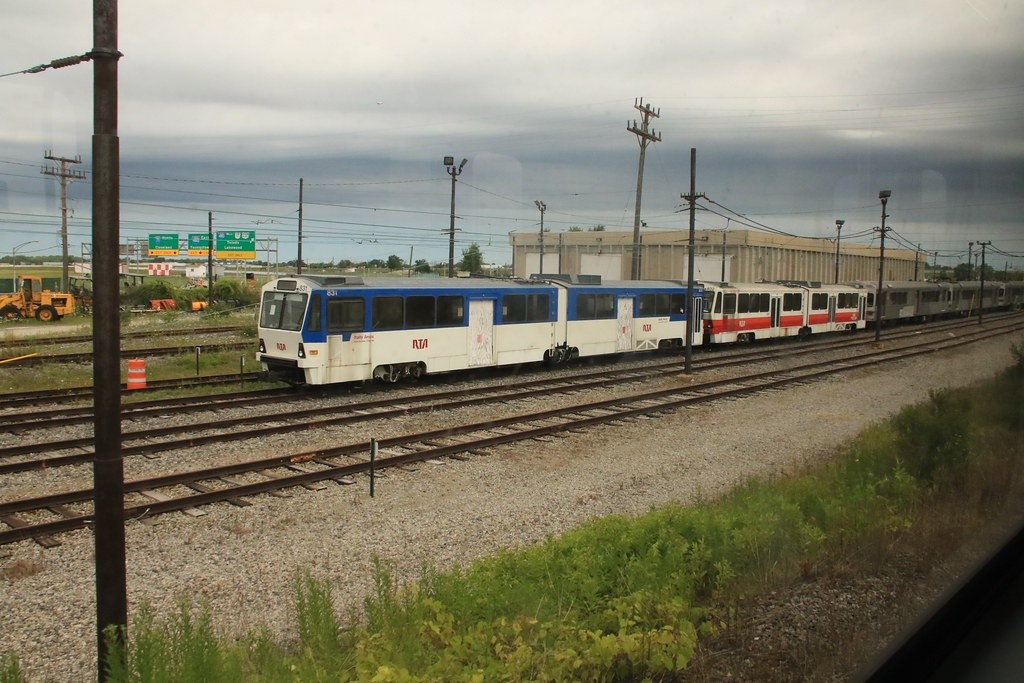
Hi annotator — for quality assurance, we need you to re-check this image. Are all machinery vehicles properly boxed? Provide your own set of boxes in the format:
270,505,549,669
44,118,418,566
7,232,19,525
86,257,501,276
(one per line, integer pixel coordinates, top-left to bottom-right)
0,274,75,323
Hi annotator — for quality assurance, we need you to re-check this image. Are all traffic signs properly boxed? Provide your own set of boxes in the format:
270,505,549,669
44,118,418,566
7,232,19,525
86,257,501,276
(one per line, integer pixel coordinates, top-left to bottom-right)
215,231,256,260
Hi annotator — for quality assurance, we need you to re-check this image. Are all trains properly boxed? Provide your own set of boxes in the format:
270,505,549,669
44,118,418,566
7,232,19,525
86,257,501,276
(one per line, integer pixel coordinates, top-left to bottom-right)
254,272,1023,394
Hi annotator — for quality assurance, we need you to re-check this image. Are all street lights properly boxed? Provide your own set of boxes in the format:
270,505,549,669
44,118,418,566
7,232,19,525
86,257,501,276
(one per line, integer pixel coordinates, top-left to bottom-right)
875,188,892,341
12,241,40,292
834,219,845,284
533,200,547,274
443,155,468,277
968,242,974,281
